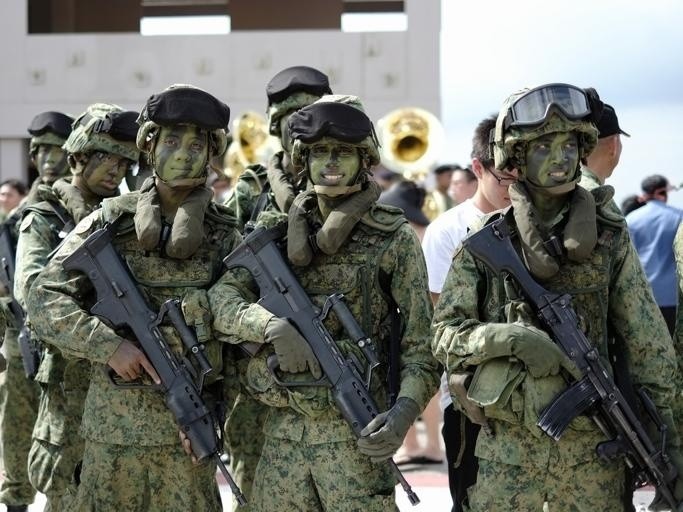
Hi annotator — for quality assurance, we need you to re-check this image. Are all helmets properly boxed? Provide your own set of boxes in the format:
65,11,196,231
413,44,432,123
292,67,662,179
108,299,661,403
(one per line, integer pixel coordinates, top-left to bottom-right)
29,132,67,155
290,94,381,168
61,103,140,162
492,84,601,168
136,84,227,158
267,93,318,137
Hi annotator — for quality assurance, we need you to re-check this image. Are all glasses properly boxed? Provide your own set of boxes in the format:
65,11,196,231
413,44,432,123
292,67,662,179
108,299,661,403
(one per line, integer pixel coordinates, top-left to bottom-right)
27,111,74,140
146,89,231,129
266,66,332,106
107,111,140,143
287,102,372,143
504,85,600,129
480,164,515,188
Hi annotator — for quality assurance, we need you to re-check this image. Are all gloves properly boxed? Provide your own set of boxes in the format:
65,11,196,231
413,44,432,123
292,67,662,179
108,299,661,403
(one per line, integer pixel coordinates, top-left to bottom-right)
358,399,417,464
511,322,582,379
266,318,322,379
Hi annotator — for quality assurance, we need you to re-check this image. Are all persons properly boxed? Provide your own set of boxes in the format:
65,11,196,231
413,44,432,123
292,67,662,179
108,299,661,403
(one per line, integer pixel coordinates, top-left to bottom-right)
11,105,152,511
206,95,445,512
429,84,683,512
25,84,242,511
0,179,25,215
206,87,683,471
0,111,77,511
223,69,336,239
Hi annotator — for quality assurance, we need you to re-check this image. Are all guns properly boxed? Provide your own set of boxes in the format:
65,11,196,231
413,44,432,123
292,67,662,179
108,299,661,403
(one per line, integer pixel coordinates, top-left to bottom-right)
222,225,421,505
0,220,41,380
463,216,682,511
62,228,247,505
